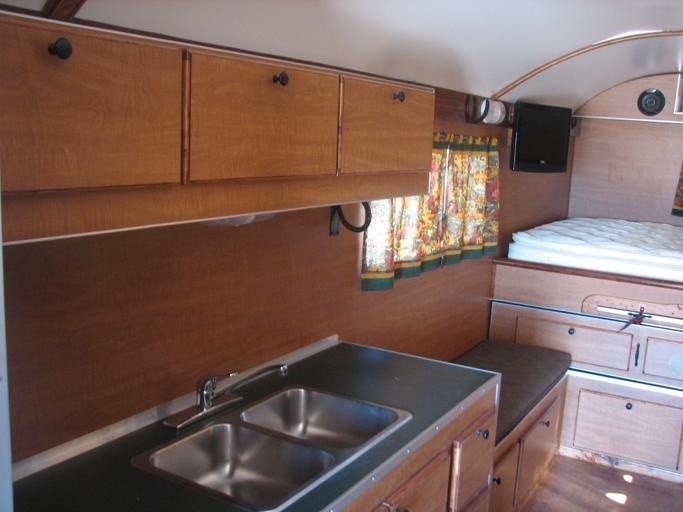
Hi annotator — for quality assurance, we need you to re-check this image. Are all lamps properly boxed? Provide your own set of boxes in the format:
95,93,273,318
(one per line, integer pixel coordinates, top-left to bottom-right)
480,99,506,125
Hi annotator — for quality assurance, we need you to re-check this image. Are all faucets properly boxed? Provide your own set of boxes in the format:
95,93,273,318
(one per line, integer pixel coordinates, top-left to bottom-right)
196,364,289,408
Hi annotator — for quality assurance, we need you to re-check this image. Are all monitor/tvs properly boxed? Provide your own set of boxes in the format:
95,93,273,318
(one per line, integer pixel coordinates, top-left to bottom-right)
510,102,572,173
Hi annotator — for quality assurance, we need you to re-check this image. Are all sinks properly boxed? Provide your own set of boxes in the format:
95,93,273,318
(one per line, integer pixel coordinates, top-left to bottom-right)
241,385,414,448
130,423,335,512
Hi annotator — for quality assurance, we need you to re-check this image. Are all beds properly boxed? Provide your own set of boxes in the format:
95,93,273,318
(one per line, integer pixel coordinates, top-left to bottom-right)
508,216,683,283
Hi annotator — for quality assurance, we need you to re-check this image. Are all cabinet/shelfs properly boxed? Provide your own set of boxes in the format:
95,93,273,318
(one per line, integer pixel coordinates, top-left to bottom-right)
489,302,682,391
560,368,683,485
341,70,437,206
341,377,567,512
0,13,184,245
188,42,338,223
